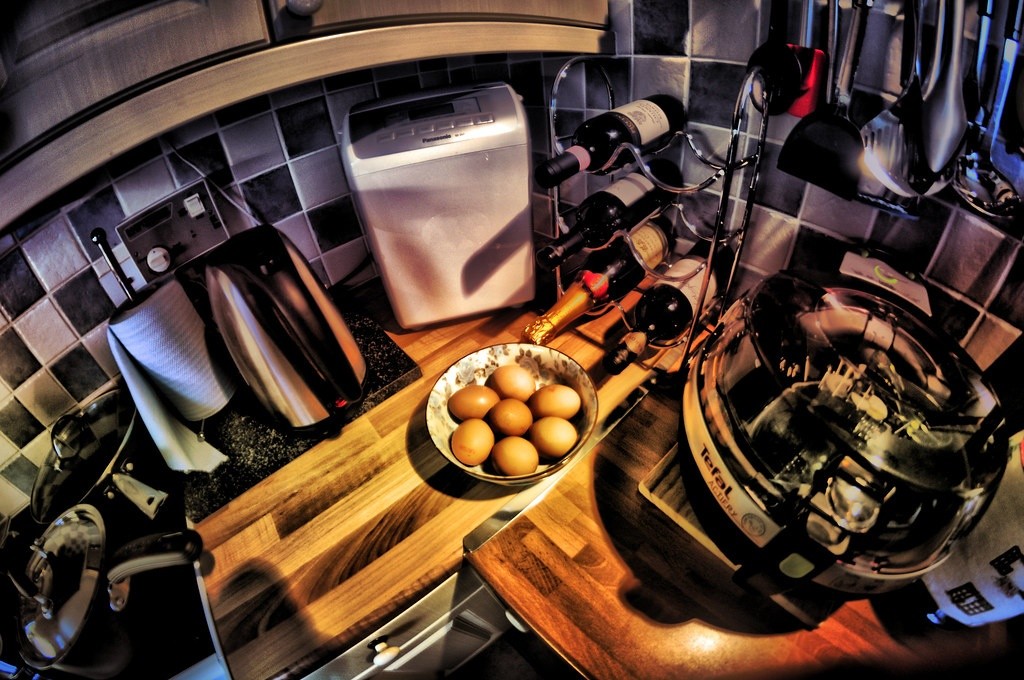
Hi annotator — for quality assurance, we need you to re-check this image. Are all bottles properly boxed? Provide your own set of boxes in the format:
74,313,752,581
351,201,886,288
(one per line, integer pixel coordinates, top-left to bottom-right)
538,94,685,189
537,160,681,268
521,210,676,343
606,239,735,373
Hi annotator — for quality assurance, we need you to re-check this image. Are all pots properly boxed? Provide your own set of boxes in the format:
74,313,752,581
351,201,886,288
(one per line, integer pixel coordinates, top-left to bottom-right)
27,387,168,521
8,502,204,675
682,273,1012,607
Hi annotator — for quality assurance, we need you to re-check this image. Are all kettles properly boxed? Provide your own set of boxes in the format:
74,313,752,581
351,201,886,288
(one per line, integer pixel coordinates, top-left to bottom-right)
202,225,368,433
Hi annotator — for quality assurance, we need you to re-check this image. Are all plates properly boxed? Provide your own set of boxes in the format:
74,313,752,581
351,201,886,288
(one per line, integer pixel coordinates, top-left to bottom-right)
425,342,598,490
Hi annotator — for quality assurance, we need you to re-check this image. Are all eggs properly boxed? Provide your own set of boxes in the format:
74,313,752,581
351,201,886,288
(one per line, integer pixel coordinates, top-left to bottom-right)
447,365,580,476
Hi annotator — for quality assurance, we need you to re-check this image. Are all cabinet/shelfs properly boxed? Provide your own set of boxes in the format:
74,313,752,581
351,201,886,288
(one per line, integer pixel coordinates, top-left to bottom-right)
184,266,1024,680
0,0,616,226
